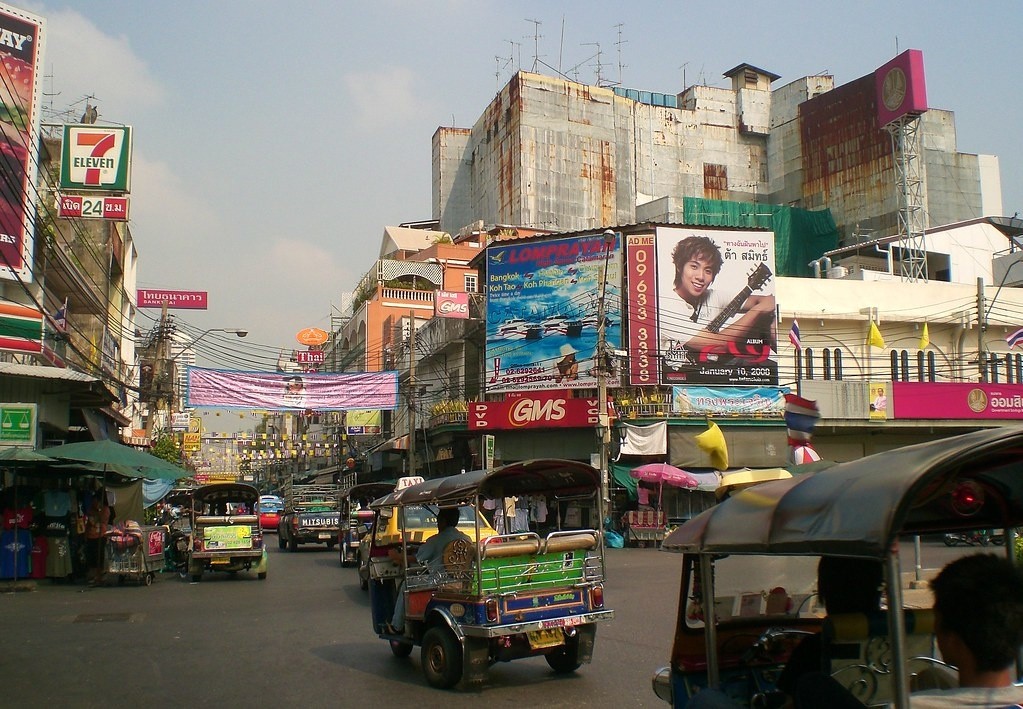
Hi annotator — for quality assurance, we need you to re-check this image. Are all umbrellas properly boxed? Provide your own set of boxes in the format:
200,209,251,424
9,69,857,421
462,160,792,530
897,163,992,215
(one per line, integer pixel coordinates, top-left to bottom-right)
0,440,194,583
630,462,698,508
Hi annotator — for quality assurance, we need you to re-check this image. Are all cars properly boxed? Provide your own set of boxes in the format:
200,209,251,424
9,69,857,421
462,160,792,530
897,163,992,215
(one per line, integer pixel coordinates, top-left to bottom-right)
253,494,285,530
355,475,505,591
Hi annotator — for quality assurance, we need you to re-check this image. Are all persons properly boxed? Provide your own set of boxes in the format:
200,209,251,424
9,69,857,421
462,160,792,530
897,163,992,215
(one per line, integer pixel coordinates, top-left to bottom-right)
86,494,111,578
553,341,619,382
657,235,778,384
775,392,786,408
356,498,368,511
873,388,886,412
215,502,228,517
928,554,1023,709
636,480,657,511
776,553,912,709
376,505,473,635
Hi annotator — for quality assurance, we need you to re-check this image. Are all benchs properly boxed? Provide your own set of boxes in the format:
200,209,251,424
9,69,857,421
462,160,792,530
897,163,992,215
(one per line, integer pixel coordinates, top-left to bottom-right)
351,507,376,526
194,513,258,541
433,523,602,599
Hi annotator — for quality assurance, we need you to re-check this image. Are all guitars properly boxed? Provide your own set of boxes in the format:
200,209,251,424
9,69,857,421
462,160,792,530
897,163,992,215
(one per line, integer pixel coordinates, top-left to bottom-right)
662,261,772,373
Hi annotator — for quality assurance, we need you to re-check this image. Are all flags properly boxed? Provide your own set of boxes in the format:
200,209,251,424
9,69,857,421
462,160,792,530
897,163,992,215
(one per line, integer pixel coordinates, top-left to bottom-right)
789,318,801,351
865,320,885,349
920,323,930,351
1005,327,1023,349
696,421,730,471
784,393,822,449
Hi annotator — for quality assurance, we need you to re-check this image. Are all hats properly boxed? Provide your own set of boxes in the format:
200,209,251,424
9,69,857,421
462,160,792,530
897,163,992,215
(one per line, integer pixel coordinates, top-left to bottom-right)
557,344,577,362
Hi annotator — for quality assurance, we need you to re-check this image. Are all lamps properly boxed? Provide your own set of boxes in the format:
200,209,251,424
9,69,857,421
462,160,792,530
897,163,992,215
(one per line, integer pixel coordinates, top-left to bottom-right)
1004,326,1007,333
817,319,824,327
914,323,919,330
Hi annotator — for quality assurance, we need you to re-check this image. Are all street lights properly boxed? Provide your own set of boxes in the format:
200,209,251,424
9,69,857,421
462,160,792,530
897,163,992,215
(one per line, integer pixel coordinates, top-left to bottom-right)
594,228,615,546
143,328,248,452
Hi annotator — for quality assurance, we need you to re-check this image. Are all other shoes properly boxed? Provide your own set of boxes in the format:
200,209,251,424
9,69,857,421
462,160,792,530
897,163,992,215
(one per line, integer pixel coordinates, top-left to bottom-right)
385,619,397,634
89,577,97,584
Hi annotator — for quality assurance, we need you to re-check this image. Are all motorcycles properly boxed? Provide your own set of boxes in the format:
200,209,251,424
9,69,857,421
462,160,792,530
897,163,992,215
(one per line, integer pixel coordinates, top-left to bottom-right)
339,481,401,569
361,455,613,691
186,482,269,583
650,424,1022,709
153,507,188,573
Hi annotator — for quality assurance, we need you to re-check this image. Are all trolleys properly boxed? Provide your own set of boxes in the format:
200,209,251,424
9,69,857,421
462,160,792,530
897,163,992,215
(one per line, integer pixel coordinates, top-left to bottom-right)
105,532,160,586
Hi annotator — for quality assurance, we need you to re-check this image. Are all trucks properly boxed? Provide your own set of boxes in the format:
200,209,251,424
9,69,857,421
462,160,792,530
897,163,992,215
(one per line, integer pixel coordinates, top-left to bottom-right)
276,473,343,552
